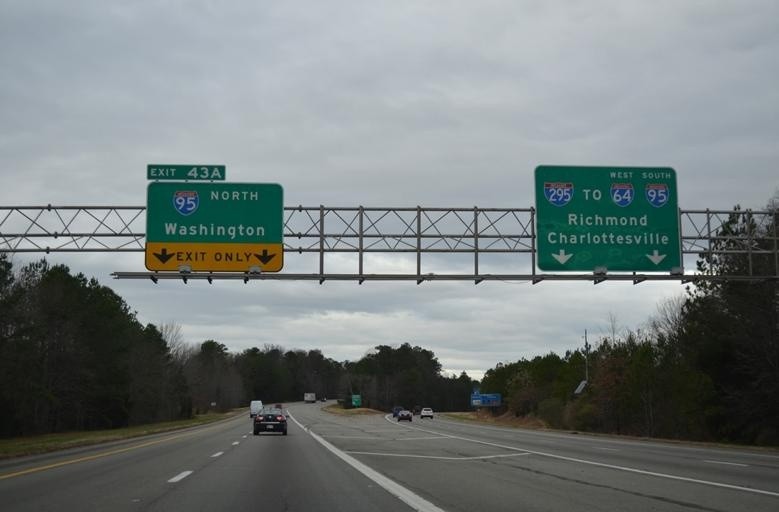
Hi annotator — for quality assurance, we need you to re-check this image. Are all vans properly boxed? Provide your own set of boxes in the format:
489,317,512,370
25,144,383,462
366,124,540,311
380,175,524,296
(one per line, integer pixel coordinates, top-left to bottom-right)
303,392,316,404
250,400,265,418
413,406,433,419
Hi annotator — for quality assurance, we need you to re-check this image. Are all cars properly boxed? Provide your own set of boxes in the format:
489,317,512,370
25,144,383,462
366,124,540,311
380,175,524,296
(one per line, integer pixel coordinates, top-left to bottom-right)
393,406,412,422
254,404,289,435
